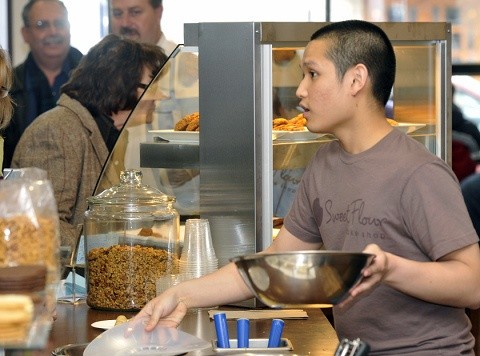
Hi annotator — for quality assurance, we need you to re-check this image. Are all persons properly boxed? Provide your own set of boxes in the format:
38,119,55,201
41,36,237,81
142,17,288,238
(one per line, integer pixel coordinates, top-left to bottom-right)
124,20,480,356
0,0,303,264
451,75,480,248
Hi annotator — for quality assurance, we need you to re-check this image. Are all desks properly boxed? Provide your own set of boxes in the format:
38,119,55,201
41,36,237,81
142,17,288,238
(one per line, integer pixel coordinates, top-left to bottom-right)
5,296,343,356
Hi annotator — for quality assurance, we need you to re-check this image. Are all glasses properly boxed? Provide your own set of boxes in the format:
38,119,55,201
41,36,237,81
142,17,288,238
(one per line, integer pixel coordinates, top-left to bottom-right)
29,19,69,31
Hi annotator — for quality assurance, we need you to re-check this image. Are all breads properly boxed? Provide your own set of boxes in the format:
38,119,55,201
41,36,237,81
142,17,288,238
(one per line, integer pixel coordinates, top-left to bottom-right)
175,111,199,131
0,294,35,344
273,113,306,131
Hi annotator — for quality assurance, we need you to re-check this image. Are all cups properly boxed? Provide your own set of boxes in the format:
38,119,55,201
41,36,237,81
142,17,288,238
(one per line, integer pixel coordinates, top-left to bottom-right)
178,219,219,312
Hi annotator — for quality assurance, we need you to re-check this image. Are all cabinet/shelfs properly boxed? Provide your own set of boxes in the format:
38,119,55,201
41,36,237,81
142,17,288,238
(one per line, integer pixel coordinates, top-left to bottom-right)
58,20,454,309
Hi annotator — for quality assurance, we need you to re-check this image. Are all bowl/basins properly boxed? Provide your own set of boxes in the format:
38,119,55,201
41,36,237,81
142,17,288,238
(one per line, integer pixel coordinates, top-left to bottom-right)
229,250,376,308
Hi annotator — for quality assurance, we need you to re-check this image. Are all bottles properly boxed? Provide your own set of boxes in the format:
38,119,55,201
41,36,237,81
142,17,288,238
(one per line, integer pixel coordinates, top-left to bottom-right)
83,169,180,310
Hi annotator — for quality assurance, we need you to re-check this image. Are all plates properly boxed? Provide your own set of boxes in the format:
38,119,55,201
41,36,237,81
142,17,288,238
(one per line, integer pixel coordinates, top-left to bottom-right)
147,129,199,143
272,127,328,140
391,123,426,134
